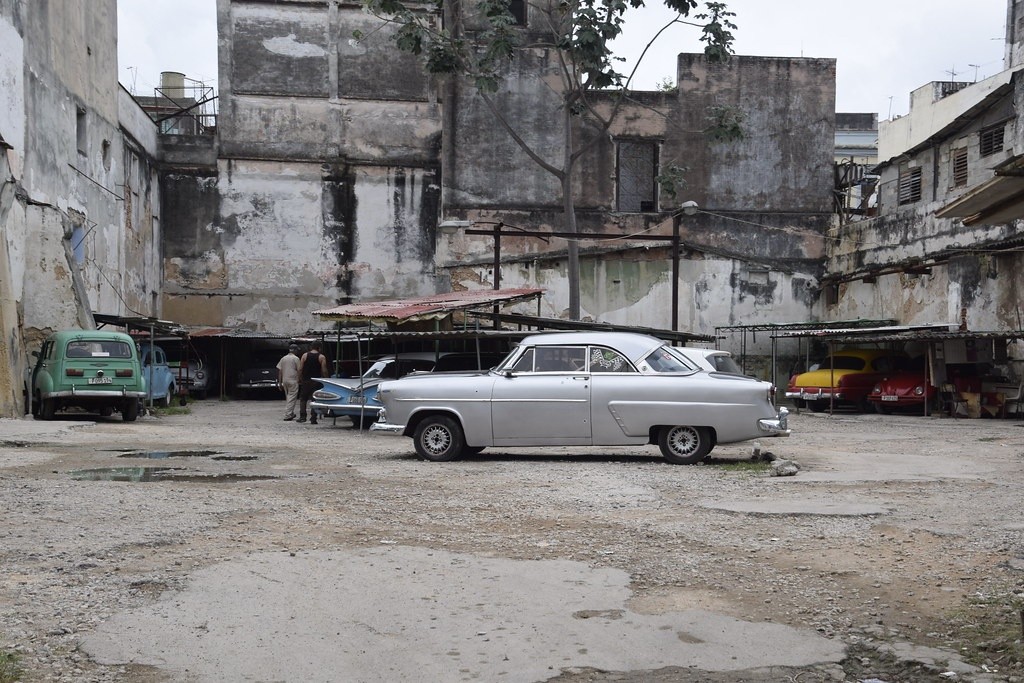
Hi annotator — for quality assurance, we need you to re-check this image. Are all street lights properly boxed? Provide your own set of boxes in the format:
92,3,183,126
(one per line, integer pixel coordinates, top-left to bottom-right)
438,221,550,367
672,200,699,346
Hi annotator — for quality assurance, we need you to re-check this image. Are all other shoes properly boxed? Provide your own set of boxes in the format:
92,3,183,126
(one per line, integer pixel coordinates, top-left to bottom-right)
284,414,296,421
311,421,317,424
296,418,306,422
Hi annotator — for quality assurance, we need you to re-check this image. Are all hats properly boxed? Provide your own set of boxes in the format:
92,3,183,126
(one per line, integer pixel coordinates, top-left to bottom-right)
289,344,301,351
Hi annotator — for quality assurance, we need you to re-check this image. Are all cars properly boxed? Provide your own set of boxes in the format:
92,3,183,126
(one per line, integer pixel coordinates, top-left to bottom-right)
309,350,497,430
235,353,283,400
370,332,791,464
31,330,216,421
785,347,935,417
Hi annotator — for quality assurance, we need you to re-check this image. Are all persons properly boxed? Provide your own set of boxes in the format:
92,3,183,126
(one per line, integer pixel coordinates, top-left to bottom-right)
296,340,328,424
275,344,300,421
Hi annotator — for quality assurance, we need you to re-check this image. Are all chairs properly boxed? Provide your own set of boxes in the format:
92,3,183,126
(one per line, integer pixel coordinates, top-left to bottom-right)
940,381,969,421
1002,379,1024,421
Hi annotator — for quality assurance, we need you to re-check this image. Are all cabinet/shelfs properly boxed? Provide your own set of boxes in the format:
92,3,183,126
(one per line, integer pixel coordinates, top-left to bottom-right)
992,384,1024,413
